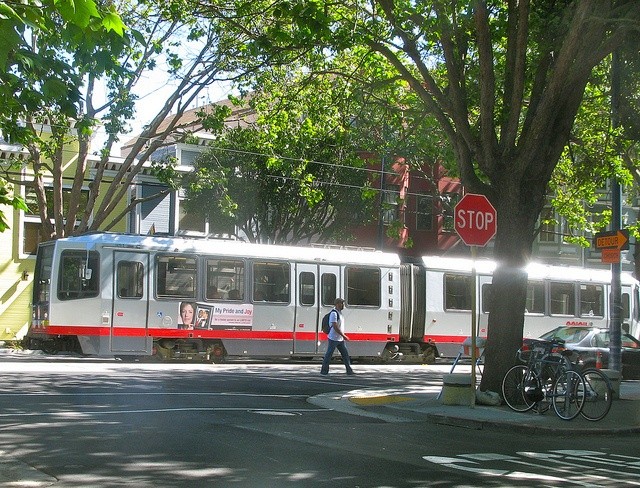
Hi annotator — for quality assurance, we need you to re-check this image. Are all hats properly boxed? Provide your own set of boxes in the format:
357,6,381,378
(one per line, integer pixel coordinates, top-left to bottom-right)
334,298,346,304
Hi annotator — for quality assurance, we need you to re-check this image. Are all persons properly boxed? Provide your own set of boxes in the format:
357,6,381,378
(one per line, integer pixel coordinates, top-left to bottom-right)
195,310,203,327
320,298,356,376
180,302,196,325
198,311,210,328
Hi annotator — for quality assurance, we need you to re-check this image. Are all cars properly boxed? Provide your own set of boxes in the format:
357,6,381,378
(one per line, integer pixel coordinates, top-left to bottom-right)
523,325,640,380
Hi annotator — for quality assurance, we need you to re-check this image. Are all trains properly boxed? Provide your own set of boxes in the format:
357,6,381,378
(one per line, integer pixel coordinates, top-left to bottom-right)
20,231,640,365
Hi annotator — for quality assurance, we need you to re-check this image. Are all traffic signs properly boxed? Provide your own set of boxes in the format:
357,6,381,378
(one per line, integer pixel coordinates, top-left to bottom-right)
601,249,620,263
595,230,629,250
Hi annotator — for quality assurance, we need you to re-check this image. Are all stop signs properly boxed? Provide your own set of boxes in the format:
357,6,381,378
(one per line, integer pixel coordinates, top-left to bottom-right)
454,192,497,247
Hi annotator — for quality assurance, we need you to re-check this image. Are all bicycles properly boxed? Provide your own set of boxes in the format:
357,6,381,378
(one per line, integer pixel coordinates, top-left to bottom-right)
521,338,612,422
501,339,586,421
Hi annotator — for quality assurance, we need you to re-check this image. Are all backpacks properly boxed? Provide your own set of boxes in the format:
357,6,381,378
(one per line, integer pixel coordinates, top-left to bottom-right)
321,310,339,334
